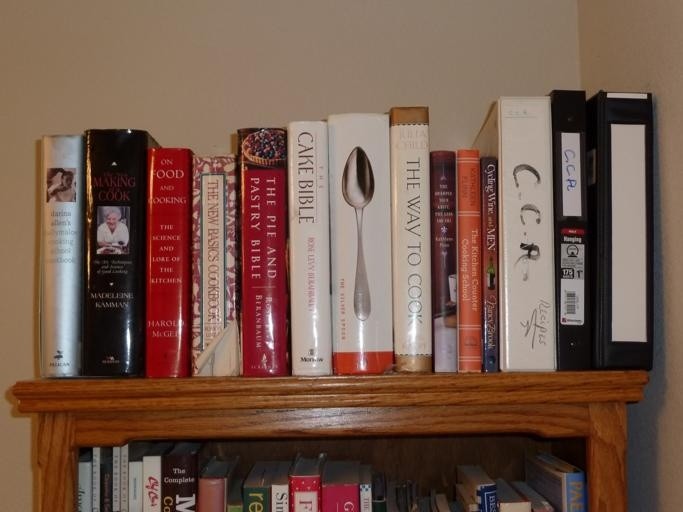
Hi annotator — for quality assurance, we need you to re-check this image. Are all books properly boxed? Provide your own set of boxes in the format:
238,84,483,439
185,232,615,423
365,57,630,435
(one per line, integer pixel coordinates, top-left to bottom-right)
78,445,389,512
38,106,433,377
433,88,659,374
385,451,585,512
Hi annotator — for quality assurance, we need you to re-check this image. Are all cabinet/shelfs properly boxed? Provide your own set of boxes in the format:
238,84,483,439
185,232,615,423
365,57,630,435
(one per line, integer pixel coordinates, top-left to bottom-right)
8,370,652,512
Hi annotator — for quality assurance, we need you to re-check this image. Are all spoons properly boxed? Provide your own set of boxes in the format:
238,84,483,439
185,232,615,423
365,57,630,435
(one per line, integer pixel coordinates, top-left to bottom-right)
340,144,374,322
